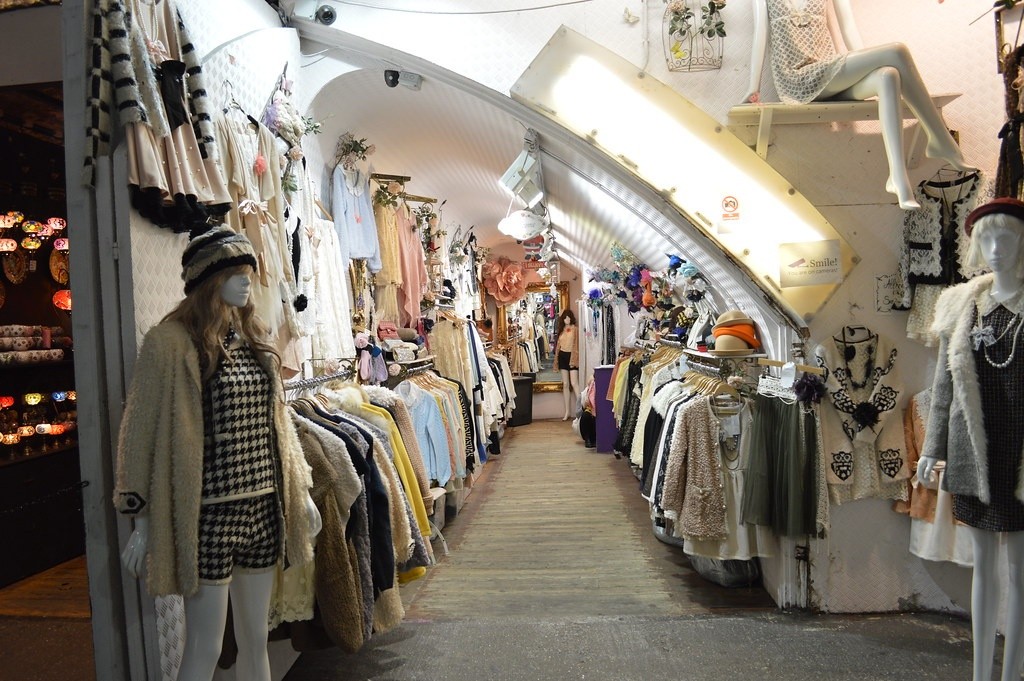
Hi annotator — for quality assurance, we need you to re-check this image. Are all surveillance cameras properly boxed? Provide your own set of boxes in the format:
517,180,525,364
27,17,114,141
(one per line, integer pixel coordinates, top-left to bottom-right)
384,70,399,88
318,4,336,25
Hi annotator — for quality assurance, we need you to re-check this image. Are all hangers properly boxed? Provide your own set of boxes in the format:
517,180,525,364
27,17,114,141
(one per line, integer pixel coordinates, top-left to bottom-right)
340,152,418,225
653,339,825,423
922,163,977,205
222,79,260,129
432,305,477,332
281,369,363,428
393,359,461,402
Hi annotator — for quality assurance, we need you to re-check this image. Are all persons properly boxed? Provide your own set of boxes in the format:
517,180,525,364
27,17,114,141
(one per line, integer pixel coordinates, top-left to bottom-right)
552,310,579,421
915,197,1023,681
740,0,981,212
111,232,326,680
536,304,545,328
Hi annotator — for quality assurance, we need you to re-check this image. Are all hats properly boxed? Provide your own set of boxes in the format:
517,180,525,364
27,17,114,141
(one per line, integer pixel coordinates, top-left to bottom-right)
964,197,1024,237
710,310,754,334
707,335,755,356
713,323,761,350
181,220,259,295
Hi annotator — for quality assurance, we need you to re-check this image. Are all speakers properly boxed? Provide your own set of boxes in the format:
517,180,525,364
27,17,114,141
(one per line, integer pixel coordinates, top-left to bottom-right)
399,72,423,91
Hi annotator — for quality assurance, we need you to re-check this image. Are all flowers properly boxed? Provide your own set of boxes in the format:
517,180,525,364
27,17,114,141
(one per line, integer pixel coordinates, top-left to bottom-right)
666,0,728,71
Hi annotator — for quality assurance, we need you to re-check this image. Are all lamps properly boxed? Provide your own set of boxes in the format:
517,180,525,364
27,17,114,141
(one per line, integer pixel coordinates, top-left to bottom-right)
498,148,538,199
518,181,545,209
0,210,79,461
